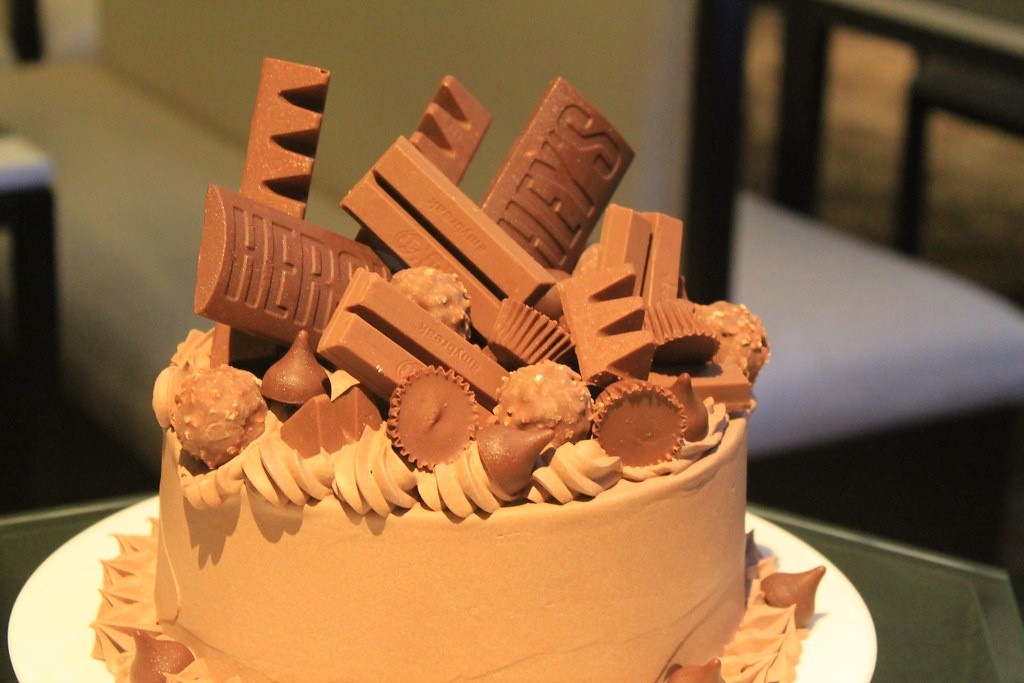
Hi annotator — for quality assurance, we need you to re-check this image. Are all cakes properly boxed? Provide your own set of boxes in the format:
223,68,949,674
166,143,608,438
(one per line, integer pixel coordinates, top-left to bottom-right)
94,56,827,683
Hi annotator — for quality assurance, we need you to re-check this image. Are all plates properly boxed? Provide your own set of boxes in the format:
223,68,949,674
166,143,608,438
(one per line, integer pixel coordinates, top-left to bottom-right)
8,498,879,683
